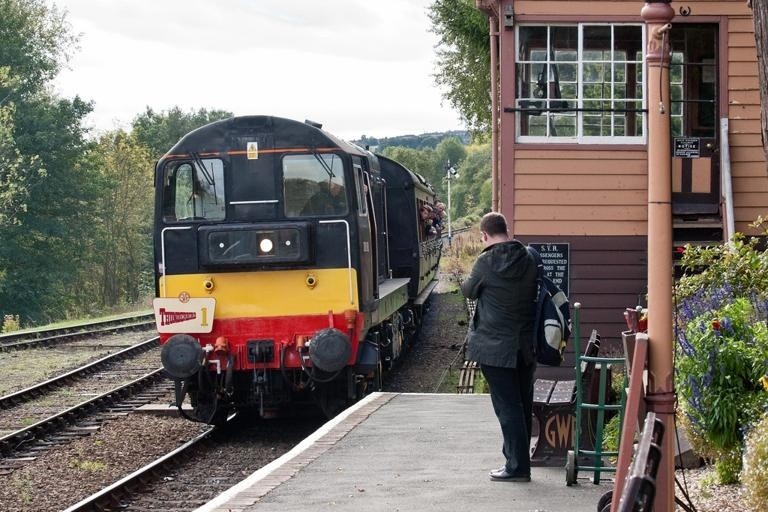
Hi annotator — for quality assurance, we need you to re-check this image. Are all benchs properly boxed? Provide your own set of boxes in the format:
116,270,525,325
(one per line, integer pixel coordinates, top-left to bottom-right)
529,327,600,466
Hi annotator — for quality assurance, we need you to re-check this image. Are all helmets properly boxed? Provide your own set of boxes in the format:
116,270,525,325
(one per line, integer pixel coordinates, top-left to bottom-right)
331,176,344,187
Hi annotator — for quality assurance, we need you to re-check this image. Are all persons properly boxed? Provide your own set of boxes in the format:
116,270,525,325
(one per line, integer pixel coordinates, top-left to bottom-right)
419,199,447,236
460,211,544,482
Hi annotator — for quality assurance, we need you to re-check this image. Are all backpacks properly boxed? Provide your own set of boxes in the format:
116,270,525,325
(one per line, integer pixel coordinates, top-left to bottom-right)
526,247,572,367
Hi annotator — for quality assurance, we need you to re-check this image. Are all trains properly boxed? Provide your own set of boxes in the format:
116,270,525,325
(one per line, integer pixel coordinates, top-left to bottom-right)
153,115,443,426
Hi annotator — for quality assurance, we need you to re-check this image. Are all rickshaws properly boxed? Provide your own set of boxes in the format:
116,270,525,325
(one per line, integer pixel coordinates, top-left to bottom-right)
564,302,629,486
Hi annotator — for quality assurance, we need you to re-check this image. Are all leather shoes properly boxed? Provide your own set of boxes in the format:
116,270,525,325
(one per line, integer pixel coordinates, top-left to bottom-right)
490,465,531,482
